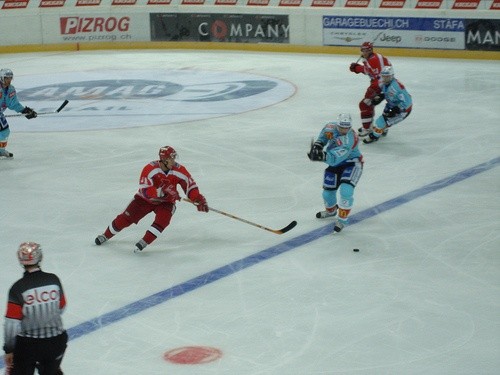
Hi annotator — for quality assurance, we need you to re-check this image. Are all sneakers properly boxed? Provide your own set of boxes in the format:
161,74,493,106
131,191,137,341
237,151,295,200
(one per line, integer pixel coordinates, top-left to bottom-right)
362,129,389,144
316,204,338,220
358,124,375,138
132,238,147,256
93,234,107,246
333,221,346,234
0,150,14,160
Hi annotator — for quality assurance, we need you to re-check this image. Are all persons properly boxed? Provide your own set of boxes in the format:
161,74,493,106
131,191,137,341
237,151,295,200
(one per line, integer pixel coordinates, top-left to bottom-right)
93,146,209,255
307,113,364,234
363,65,412,145
350,42,392,136
0,67,38,161
3,241,69,375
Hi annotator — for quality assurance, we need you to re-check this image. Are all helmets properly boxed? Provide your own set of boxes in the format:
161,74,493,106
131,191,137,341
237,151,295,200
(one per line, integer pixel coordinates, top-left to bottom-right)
0,68,13,83
17,241,43,265
337,112,352,129
159,146,177,160
380,66,394,76
361,42,373,53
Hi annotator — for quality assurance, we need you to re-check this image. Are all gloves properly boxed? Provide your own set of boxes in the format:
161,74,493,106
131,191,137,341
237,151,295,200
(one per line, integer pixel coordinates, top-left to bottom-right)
156,184,181,203
188,187,209,213
363,98,373,106
350,63,361,74
307,140,327,162
20,106,37,120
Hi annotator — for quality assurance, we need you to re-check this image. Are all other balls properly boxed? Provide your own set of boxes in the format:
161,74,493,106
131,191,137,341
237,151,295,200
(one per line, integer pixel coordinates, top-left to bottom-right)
354,249,358,251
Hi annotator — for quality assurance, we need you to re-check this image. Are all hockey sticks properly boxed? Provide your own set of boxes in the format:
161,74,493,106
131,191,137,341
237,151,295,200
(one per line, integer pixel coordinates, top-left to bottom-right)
2,98,69,118
355,27,393,65
310,137,314,152
178,198,297,234
